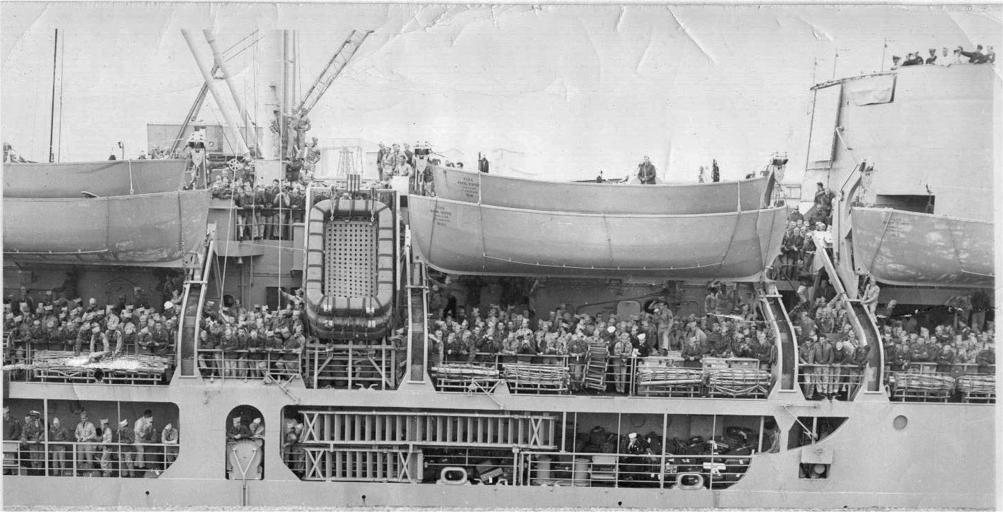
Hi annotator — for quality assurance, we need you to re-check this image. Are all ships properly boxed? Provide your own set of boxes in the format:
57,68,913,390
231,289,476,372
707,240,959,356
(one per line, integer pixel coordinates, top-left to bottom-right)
2,25,997,512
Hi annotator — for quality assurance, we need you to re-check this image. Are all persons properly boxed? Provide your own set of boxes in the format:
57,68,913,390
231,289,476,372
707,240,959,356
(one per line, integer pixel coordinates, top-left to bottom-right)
213,175,346,241
697,159,720,183
861,275,995,403
196,287,408,390
227,416,265,441
3,142,22,163
427,275,777,399
185,165,201,190
108,155,116,160
481,152,488,173
768,183,871,400
280,417,305,477
891,44,996,68
3,405,179,478
138,144,173,160
377,140,464,198
634,155,658,185
271,110,321,180
3,272,185,385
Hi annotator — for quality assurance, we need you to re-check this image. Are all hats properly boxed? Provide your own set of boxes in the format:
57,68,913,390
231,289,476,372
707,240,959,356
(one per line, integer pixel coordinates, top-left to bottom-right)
287,423,303,430
100,418,109,425
120,419,128,427
4,298,174,335
233,416,241,422
165,423,172,432
3,406,10,413
30,410,40,416
253,418,261,424
53,417,59,423
250,304,301,337
